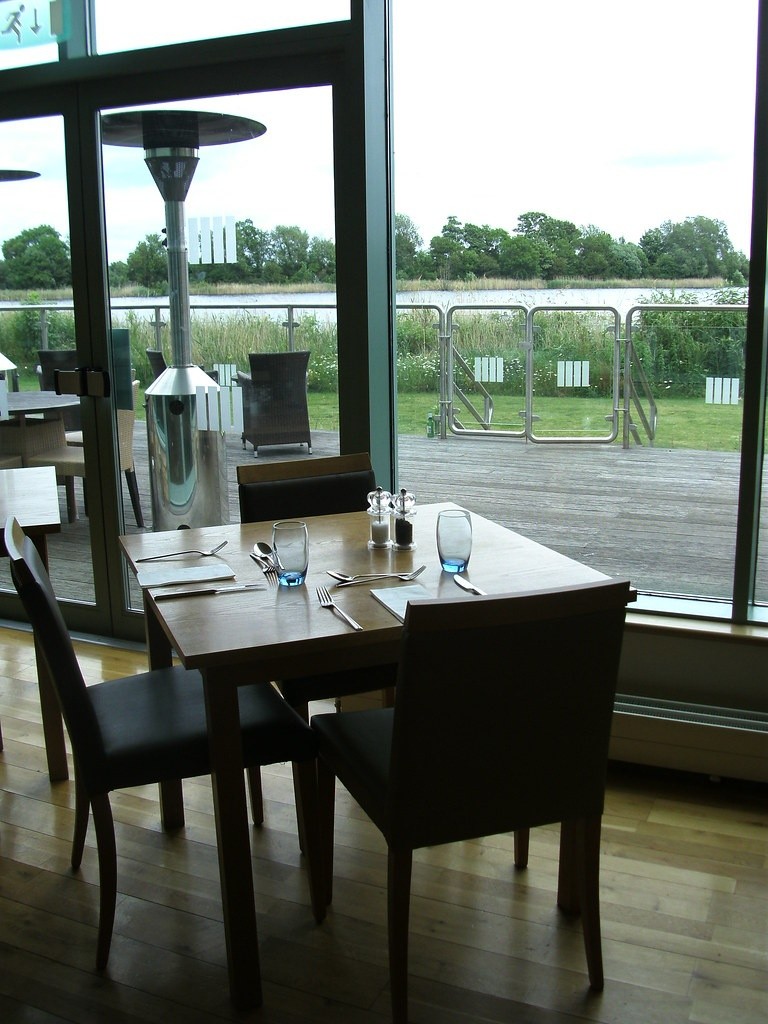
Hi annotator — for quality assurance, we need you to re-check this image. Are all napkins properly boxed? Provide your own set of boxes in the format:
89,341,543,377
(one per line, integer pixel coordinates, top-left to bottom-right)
372,585,440,624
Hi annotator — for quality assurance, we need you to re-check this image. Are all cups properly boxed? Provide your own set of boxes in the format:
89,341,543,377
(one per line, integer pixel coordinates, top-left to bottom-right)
435,509,473,572
271,520,309,587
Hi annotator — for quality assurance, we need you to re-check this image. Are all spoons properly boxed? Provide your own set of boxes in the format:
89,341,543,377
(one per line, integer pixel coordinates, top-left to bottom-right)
253,541,280,566
326,569,411,582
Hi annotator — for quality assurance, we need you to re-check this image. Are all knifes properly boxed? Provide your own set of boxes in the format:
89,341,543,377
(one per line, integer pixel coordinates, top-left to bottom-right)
154,583,267,601
454,574,487,596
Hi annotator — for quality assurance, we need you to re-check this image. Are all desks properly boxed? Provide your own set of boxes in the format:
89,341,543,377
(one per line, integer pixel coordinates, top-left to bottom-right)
119,501,638,1024
8,389,80,453
0,465,62,793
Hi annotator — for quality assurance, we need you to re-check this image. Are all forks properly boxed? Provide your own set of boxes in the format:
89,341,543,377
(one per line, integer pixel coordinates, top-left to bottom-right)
335,565,427,589
250,551,277,574
316,586,362,630
134,541,230,563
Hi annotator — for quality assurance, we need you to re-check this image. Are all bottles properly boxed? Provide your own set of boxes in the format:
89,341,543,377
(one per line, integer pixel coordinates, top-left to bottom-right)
365,486,395,550
391,489,419,551
426,412,440,438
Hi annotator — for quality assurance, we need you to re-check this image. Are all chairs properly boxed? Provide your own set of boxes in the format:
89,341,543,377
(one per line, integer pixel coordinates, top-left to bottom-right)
231,348,312,458
235,453,378,830
64,379,140,445
144,349,224,446
54,367,83,446
26,410,144,530
309,578,632,1024
5,516,310,971
